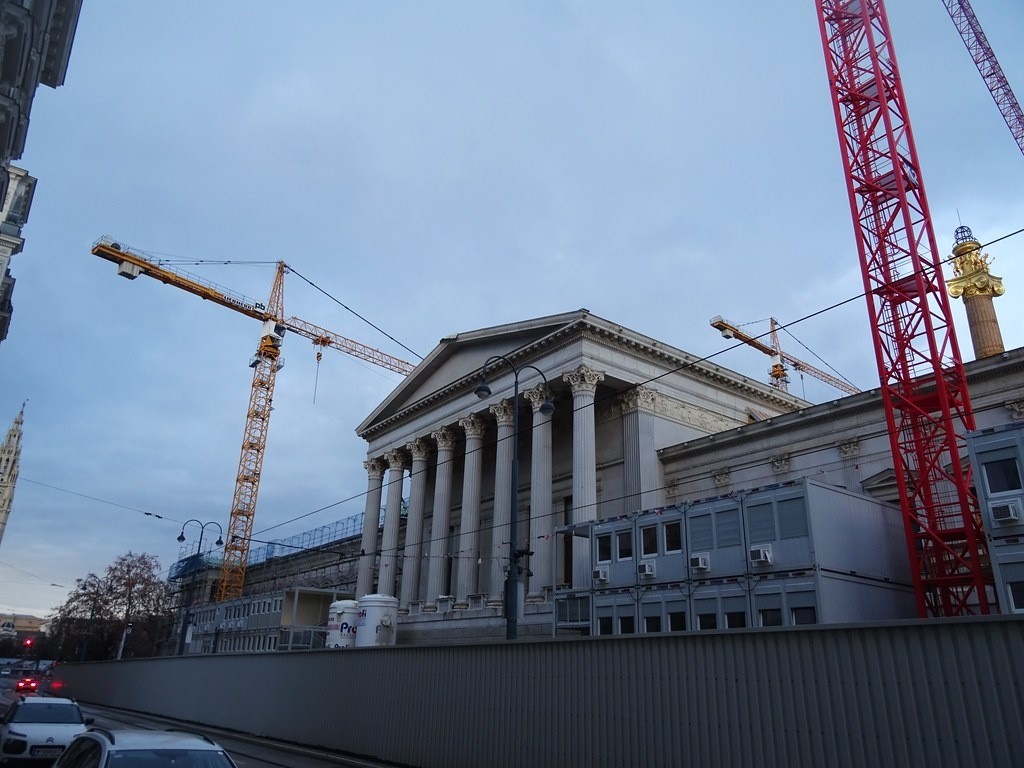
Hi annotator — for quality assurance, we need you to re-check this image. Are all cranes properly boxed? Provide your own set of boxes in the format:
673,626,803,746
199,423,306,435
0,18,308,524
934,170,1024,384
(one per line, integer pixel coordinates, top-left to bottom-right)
90,235,424,602
709,313,862,396
819,0,1023,620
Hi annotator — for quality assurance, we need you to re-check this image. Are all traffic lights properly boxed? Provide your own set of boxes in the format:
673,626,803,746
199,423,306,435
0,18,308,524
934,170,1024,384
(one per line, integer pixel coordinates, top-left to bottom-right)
25,638,32,646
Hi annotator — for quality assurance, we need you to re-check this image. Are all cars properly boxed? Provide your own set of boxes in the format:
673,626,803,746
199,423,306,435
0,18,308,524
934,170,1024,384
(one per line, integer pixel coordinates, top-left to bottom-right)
0,694,95,768
14,673,39,692
2,669,10,677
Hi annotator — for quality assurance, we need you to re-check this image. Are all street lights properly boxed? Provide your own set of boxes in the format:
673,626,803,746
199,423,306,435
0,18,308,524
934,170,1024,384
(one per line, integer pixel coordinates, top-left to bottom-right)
473,355,557,640
177,519,224,655
79,575,114,662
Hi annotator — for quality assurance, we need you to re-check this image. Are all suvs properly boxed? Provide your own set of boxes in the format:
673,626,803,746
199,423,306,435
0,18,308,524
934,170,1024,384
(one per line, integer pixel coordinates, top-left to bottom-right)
51,726,237,768
